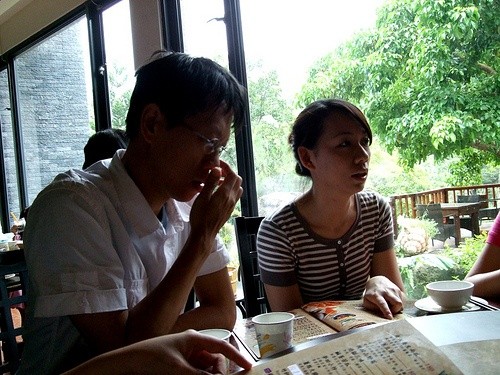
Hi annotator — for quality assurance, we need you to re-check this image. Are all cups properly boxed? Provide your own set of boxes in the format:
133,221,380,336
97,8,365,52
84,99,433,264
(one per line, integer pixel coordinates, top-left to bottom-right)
8,242,17,250
252,312,295,358
198,329,231,369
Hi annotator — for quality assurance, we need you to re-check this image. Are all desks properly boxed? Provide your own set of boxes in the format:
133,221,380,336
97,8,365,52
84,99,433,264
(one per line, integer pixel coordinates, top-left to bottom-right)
413,202,480,246
229,299,500,375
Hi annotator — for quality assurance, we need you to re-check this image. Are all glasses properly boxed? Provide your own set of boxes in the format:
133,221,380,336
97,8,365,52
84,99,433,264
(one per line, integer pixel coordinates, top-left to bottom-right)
161,109,226,156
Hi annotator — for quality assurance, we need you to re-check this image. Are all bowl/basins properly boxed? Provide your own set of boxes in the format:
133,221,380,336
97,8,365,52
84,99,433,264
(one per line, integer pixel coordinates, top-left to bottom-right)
426,280,474,307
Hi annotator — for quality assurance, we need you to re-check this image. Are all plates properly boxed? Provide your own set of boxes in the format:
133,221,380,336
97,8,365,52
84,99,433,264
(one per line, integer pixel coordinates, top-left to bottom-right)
414,297,480,312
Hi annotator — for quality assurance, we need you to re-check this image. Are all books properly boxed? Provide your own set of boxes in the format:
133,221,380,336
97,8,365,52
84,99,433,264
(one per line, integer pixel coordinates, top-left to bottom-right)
232,320,465,374
232,300,406,362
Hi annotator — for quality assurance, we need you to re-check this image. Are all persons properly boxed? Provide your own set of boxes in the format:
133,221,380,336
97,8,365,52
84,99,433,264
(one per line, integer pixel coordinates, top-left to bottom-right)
256,99,405,320
22,49,244,374
63,329,252,375
463,210,500,296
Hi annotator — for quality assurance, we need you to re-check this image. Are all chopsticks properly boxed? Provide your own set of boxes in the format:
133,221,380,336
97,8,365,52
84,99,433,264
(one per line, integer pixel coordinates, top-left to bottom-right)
469,298,500,312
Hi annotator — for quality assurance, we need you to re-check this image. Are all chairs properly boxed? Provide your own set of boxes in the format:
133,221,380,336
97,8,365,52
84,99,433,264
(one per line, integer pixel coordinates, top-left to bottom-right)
415,203,456,247
0,249,29,375
456,195,480,236
